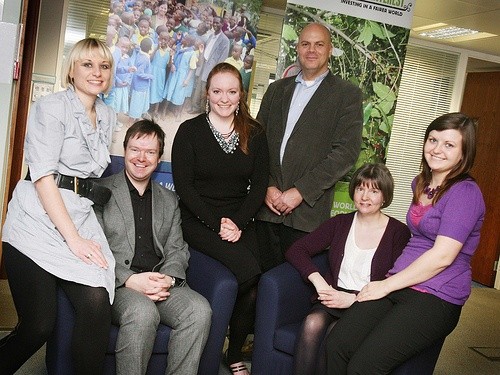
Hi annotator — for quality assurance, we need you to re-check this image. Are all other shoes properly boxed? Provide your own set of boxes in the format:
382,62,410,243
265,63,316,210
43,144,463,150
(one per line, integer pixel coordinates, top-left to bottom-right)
240,340,254,361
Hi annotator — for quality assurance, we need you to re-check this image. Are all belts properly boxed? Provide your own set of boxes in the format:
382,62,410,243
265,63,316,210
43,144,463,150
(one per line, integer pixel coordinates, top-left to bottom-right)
24,166,113,206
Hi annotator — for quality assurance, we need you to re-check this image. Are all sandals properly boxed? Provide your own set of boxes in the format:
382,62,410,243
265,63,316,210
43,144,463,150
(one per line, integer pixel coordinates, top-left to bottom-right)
223,349,250,375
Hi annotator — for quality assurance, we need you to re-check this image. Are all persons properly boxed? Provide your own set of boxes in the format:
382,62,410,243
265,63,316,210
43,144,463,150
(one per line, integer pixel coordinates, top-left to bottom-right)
286,163,414,375
172,62,274,375
254,22,364,266
92,119,212,375
315,112,485,375
104,1,257,119
1,38,117,374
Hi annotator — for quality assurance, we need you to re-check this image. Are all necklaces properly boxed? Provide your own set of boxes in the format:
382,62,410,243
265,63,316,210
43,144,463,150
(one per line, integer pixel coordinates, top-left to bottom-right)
205,117,242,155
424,186,440,199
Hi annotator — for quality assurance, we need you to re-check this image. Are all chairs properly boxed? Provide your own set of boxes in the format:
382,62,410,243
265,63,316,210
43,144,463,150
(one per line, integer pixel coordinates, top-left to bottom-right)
45,242,239,375
248,249,445,375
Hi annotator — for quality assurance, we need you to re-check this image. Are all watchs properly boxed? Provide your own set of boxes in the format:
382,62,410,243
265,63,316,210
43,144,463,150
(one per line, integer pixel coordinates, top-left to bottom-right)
171,277,176,289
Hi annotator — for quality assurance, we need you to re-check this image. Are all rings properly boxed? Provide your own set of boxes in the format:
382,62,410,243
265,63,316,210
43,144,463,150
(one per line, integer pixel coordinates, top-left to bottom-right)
86,254,92,258
